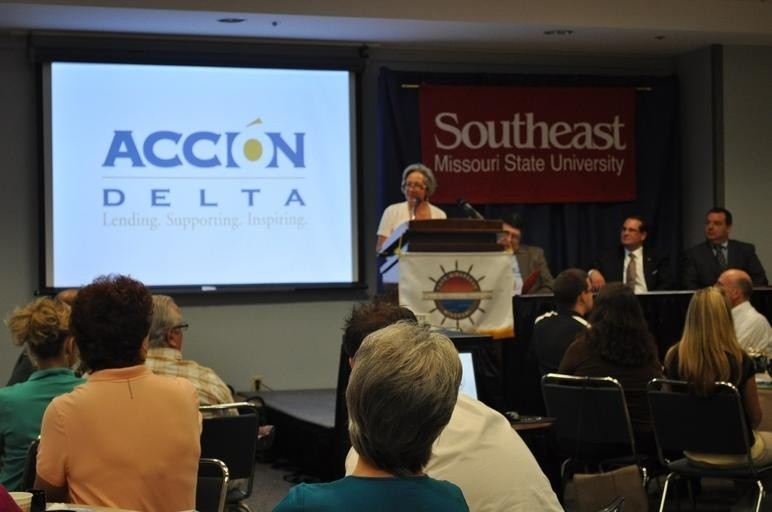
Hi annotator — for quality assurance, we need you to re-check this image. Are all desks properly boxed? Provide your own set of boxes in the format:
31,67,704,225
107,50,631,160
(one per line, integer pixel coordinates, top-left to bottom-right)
500,287,772,412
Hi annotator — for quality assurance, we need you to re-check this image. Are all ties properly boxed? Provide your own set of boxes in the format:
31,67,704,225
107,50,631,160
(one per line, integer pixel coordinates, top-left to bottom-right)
714,243,727,270
626,253,636,291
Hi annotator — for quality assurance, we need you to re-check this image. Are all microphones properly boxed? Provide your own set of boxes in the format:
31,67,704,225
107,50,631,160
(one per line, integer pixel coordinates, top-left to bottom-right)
410,196,422,220
457,198,484,219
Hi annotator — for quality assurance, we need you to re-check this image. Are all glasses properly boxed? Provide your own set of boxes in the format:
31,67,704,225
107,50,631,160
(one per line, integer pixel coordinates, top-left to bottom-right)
168,322,189,332
405,183,427,189
586,288,596,293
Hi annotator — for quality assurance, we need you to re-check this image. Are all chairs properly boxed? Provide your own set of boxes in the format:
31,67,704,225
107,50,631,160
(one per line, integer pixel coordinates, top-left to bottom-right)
646,377,764,512
198,402,261,512
197,457,231,512
541,372,648,490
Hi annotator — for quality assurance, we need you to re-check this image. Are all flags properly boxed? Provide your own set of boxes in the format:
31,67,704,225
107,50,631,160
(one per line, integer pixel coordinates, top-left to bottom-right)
398,253,515,339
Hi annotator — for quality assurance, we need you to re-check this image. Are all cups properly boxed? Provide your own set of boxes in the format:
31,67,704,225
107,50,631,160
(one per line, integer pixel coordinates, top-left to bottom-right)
7,491,33,512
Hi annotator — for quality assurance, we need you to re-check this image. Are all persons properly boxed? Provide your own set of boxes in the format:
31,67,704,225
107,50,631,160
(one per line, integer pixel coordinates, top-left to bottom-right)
375,164,449,254
343,302,566,512
534,268,772,476
0,275,238,512
675,210,768,290
493,216,551,293
270,318,470,512
586,214,665,293
